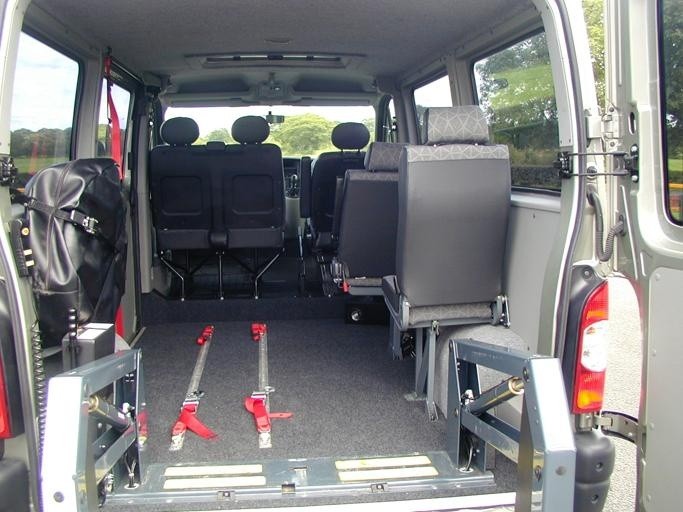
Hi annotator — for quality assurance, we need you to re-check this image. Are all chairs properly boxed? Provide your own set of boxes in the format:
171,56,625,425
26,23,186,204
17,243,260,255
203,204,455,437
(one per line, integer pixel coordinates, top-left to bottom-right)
299,121,371,251
379,106,512,402
217,115,288,299
148,117,219,300
331,140,414,297
